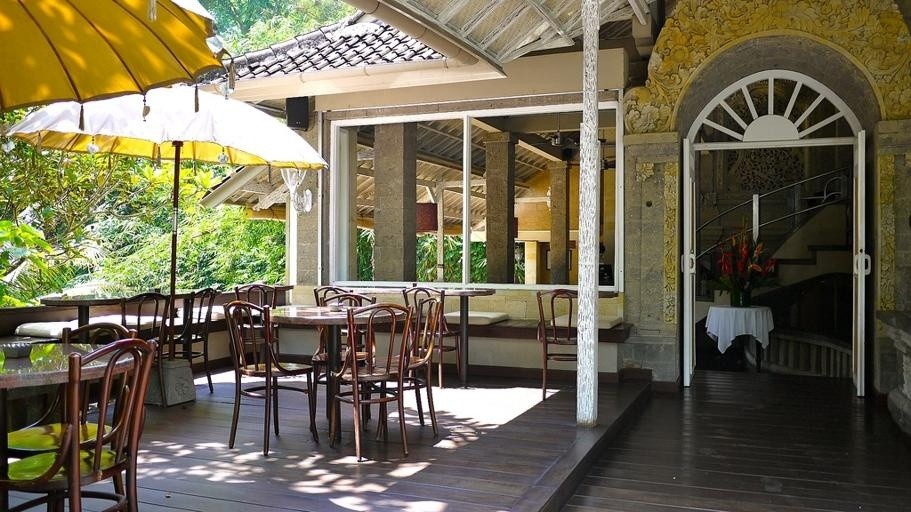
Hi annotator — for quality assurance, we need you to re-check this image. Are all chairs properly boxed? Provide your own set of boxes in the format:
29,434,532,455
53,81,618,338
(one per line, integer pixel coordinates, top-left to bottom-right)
234,284,280,366
403,287,460,388
223,300,318,455
313,292,378,432
121,292,171,408
153,288,224,394
536,290,579,400
369,296,441,441
314,286,367,354
0,339,156,512
7,322,137,512
329,303,409,462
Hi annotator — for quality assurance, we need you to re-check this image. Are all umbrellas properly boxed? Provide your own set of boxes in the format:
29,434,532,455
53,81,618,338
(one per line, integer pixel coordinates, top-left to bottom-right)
4,83,330,359
0,0,236,131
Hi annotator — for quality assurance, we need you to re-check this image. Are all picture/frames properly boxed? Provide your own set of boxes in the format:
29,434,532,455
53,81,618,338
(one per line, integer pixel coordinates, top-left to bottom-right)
546,249,571,271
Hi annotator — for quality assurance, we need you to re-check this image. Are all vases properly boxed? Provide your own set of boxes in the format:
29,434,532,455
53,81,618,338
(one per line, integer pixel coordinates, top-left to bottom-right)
730,291,752,307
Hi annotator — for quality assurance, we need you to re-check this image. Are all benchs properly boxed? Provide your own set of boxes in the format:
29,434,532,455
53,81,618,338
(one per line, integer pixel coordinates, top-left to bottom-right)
94,305,634,396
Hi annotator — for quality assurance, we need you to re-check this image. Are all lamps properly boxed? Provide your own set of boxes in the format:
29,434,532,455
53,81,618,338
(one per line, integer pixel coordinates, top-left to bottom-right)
600,144,607,170
551,112,564,147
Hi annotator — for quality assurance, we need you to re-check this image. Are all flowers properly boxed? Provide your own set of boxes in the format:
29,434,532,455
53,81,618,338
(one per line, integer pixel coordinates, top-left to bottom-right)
714,215,779,291
